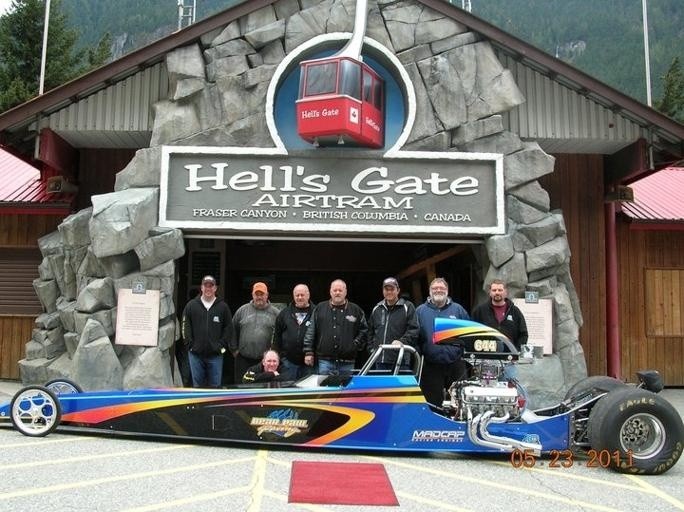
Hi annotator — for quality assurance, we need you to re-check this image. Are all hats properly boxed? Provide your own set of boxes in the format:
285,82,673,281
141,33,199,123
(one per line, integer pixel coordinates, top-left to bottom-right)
253,282,268,294
202,275,216,285
383,277,398,288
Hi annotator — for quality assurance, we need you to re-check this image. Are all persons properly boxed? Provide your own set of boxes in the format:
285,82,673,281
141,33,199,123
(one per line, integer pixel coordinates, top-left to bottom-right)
367,276,421,374
242,349,281,384
302,278,369,376
269,283,318,383
180,275,240,387
227,280,280,385
471,279,529,352
415,277,471,417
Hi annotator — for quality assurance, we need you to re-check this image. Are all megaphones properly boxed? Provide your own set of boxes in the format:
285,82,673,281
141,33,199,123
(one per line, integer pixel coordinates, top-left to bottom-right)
46,176,77,194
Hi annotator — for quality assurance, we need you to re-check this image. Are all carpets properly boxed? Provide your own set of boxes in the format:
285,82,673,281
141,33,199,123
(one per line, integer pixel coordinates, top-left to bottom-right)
286,459,399,505
